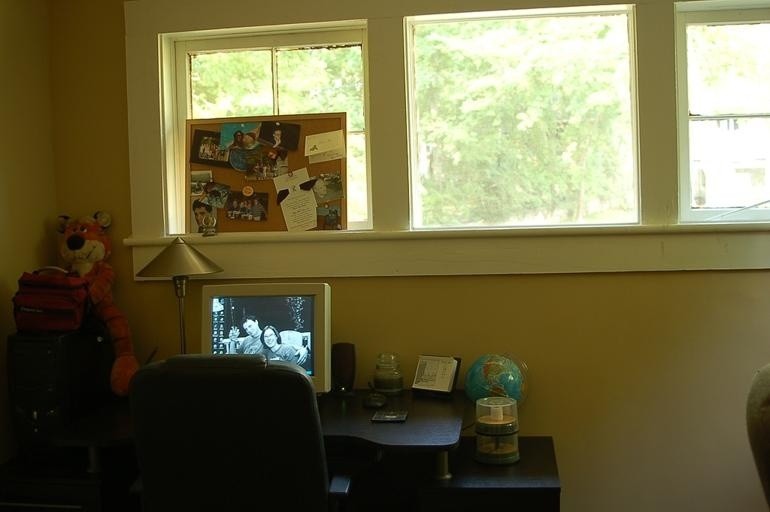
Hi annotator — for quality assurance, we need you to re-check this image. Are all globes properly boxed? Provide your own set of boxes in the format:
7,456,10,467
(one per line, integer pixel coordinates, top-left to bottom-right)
465,352,530,415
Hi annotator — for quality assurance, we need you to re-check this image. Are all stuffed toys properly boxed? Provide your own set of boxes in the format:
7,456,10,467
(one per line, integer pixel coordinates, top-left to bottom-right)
54,209,142,396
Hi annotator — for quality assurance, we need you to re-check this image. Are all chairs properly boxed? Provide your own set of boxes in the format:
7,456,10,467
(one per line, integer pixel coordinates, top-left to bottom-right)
746,364,770,510
128,354,329,512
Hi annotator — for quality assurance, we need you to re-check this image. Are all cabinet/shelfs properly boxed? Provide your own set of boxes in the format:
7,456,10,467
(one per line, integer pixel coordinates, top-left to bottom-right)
0,457,104,512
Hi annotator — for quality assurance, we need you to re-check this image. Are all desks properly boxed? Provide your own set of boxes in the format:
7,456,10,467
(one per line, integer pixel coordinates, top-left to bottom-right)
318,386,561,512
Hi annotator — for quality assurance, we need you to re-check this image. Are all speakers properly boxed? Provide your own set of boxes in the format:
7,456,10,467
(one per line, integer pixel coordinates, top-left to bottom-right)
331,342,356,392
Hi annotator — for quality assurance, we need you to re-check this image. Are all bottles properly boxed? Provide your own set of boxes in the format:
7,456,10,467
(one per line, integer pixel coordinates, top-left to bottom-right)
372,349,405,395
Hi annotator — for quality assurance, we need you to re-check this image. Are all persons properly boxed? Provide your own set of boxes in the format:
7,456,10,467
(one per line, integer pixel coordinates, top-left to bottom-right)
259,322,300,366
227,312,312,369
188,124,344,234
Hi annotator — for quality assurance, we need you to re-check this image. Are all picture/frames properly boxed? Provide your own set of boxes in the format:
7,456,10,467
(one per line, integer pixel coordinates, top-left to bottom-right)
181,112,348,232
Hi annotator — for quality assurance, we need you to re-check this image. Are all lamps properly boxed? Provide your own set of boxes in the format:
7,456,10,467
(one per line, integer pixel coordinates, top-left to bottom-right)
137,236,224,355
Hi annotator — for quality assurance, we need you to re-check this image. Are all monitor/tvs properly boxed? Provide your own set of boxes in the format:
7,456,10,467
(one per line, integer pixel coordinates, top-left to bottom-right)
200,282,331,394
